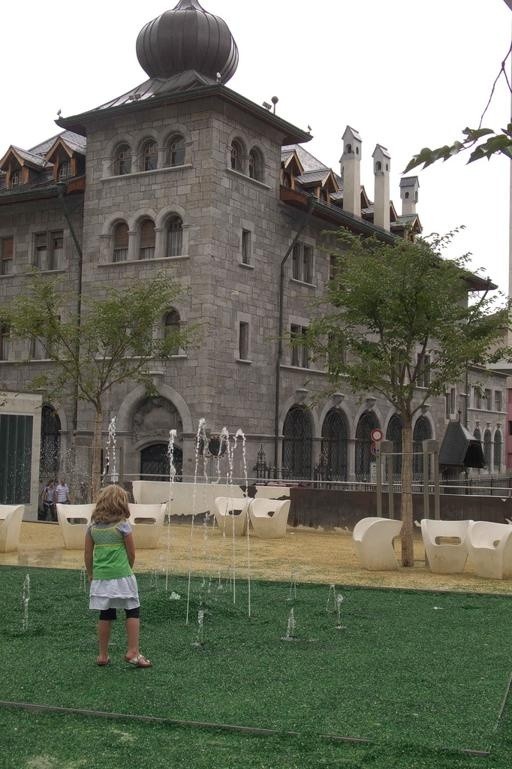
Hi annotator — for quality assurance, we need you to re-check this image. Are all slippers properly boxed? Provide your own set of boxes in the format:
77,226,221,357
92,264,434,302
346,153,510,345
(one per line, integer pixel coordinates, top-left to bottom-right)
96,655,109,665
125,654,152,666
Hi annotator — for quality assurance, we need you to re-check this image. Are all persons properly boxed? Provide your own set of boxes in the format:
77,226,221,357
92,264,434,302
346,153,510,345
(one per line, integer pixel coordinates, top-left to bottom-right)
54,478,74,521
83,485,154,670
42,479,56,521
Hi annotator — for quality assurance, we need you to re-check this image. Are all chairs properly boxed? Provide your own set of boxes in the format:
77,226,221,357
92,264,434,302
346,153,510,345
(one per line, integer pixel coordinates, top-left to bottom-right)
56,502,168,551
212,497,291,540
0,504,25,553
351,516,511,580
